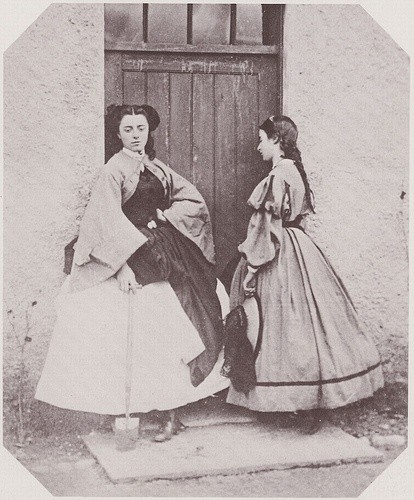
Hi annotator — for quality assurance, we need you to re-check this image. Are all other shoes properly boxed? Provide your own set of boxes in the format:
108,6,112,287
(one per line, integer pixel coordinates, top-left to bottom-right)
152,412,184,443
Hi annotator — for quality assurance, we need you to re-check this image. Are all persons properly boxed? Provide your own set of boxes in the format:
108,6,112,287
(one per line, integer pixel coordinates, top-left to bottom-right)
33,102,235,443
220,114,385,435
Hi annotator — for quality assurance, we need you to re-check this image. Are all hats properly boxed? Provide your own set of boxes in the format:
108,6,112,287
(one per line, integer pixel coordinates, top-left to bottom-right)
226,294,264,365
